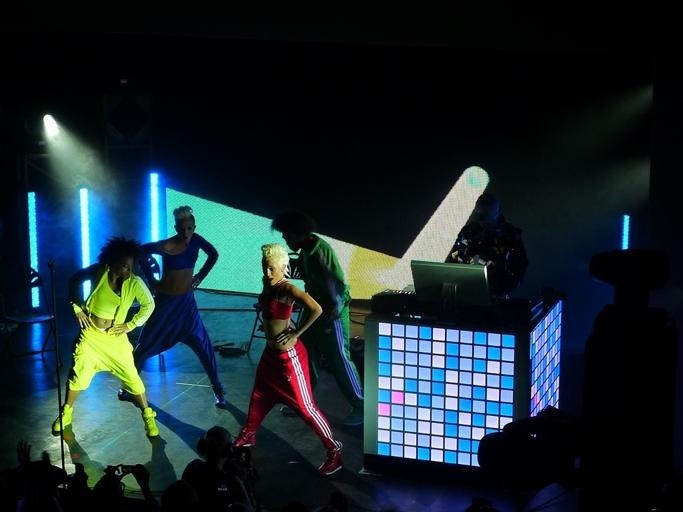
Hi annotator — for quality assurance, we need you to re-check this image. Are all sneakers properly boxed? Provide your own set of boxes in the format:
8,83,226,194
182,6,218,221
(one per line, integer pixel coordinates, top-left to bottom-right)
213,391,228,408
340,405,363,427
314,448,344,477
230,426,256,449
140,407,159,437
116,387,130,400
50,403,73,432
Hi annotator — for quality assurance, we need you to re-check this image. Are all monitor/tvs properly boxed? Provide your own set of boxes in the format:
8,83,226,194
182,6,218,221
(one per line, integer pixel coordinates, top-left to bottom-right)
410,261,491,327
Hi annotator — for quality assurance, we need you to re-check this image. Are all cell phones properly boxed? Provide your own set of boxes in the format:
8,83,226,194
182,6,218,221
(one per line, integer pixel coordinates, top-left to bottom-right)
122,465,135,473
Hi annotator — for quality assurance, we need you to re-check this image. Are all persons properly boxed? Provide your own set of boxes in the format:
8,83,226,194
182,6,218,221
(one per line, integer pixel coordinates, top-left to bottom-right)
51,236,160,439
451,193,528,297
117,206,225,408
230,243,344,476
1,426,349,512
272,210,363,428
